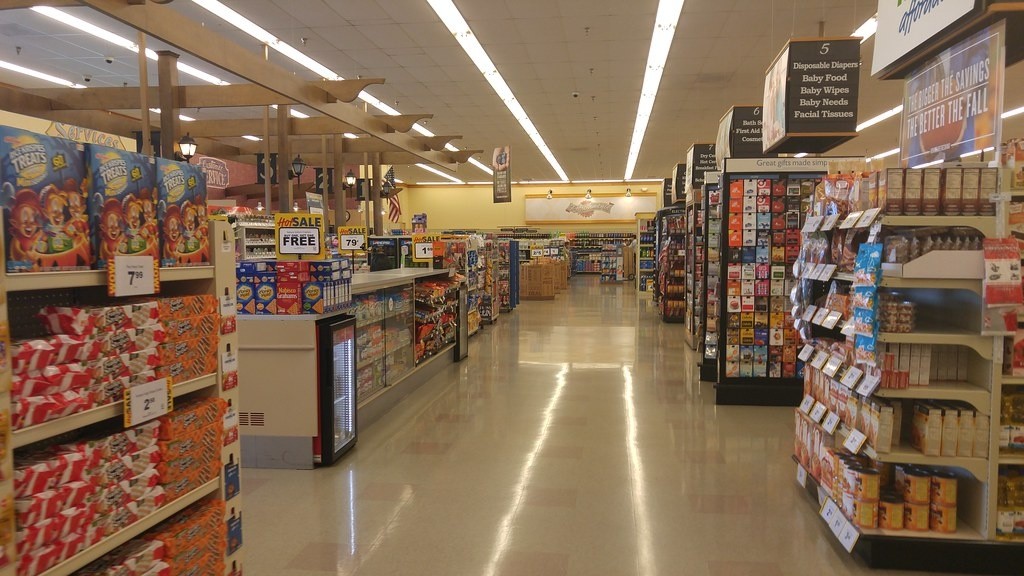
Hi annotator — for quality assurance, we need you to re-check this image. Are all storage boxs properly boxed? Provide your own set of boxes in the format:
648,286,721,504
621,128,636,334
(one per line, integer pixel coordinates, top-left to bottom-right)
0,125,351,576
795,166,997,496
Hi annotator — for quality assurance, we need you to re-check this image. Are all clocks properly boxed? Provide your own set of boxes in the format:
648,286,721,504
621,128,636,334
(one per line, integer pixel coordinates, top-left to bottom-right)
346,211,350,221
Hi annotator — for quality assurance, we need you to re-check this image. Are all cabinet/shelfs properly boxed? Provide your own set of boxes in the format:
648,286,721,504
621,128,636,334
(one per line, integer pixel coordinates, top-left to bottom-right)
635,172,827,406
792,168,1024,576
0,109,242,576
231,222,635,470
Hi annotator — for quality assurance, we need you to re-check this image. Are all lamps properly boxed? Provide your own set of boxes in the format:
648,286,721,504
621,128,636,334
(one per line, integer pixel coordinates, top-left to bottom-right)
292,201,301,211
547,190,552,198
256,202,264,211
343,168,357,190
175,131,198,163
288,152,306,188
585,189,591,199
626,188,631,197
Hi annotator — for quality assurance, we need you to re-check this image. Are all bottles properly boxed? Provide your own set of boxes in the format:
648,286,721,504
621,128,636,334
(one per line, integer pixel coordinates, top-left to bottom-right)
995,384,1024,542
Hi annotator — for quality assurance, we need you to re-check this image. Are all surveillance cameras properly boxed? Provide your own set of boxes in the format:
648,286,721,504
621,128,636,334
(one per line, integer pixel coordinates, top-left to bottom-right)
572,93,579,98
82,74,92,82
104,57,114,64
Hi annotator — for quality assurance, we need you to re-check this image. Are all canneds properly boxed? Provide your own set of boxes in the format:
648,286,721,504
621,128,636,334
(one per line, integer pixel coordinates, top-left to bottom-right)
831,452,957,534
